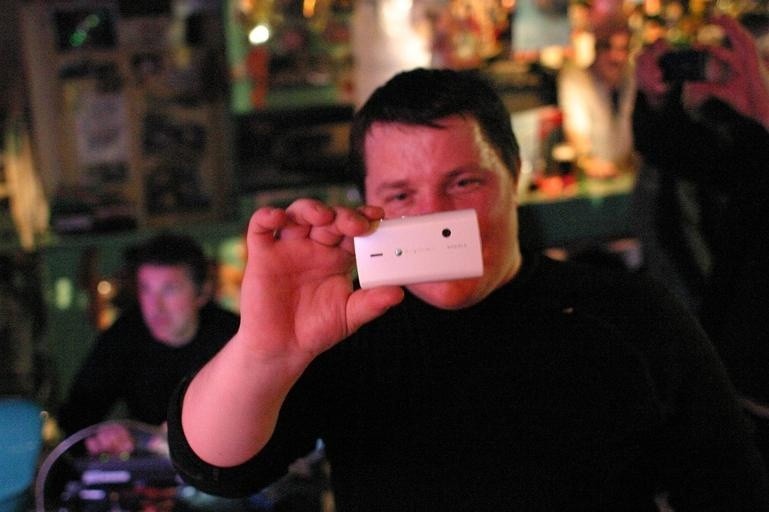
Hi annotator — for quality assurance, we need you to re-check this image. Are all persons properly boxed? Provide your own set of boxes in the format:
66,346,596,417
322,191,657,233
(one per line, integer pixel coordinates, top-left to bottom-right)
559,17,642,183
631,8,769,402
166,66,767,508
18,232,325,512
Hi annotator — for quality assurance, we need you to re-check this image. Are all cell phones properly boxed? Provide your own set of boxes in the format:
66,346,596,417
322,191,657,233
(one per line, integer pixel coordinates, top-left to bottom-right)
657,47,717,84
353,208,484,290
